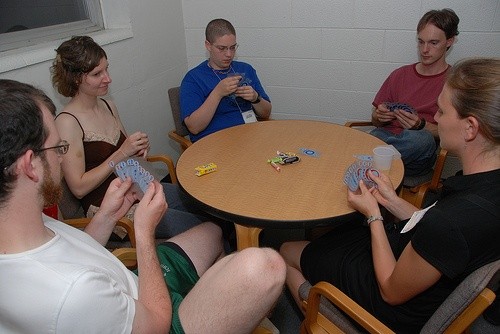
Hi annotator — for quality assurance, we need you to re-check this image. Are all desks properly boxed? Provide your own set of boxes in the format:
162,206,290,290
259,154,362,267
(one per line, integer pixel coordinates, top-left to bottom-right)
174,118,405,253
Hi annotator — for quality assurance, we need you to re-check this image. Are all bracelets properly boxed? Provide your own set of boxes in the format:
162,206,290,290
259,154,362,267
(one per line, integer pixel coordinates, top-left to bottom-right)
252,95,261,104
367,216,383,224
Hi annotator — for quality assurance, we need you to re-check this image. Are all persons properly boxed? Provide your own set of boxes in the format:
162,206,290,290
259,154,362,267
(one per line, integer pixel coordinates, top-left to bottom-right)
0,78,287,334
50,35,237,255
368,9,460,174
280,57,500,334
180,19,272,143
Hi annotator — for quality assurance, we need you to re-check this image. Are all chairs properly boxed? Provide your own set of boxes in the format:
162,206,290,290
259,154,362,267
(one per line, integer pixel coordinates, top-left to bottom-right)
111,248,281,334
345,121,448,210
167,87,192,155
297,259,500,334
59,153,179,249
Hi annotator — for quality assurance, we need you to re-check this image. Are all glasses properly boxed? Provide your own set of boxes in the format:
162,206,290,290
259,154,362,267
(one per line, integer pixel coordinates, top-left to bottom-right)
209,42,239,53
34,139,71,154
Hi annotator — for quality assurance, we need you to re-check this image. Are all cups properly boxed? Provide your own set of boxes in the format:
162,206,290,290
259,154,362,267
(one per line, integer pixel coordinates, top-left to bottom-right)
372,147,394,178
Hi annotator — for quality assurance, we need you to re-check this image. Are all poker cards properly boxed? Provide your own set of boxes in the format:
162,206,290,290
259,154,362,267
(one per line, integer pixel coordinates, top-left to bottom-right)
107,156,169,200
342,159,381,195
228,72,253,87
297,147,320,158
383,101,418,115
